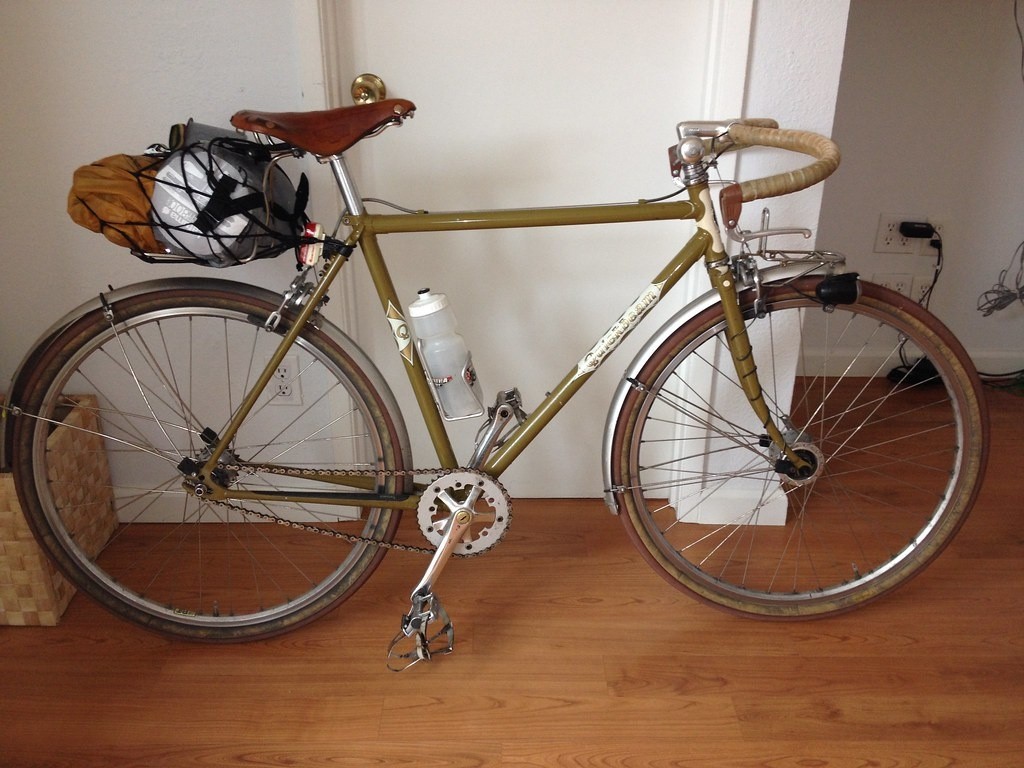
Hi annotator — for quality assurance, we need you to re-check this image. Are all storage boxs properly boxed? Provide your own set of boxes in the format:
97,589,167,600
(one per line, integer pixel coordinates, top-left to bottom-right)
0,390,118,624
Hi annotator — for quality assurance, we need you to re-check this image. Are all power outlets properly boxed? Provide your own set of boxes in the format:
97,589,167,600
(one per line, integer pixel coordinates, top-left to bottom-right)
263,354,304,407
923,214,945,255
873,212,922,255
874,272,912,299
911,276,935,303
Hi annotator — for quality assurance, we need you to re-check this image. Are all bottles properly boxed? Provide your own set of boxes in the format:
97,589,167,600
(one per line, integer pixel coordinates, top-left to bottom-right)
408,288,483,419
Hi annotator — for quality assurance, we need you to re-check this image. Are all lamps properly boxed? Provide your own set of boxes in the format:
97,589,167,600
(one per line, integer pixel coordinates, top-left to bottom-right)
815,273,863,314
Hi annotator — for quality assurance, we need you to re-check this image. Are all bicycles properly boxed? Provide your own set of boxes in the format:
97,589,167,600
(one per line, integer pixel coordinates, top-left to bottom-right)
0,98,994,661
